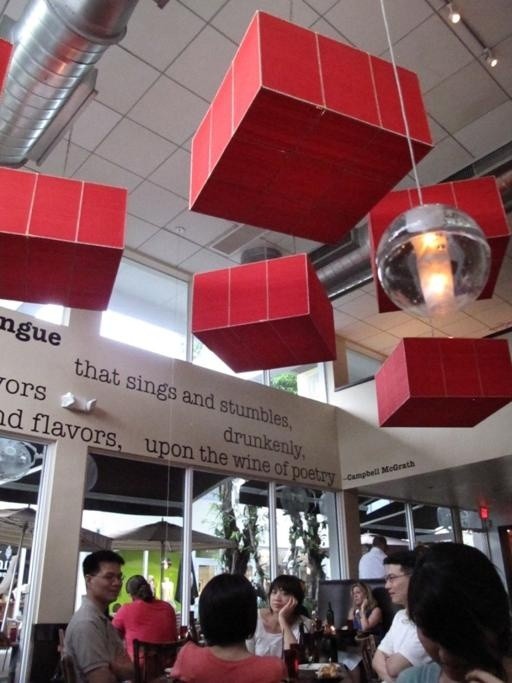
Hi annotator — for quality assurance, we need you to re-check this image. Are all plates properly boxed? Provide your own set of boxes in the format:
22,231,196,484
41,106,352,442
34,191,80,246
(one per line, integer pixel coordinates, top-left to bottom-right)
297,661,340,670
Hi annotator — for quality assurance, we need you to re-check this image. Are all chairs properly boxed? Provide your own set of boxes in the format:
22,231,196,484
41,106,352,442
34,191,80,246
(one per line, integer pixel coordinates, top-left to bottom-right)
23,617,390,683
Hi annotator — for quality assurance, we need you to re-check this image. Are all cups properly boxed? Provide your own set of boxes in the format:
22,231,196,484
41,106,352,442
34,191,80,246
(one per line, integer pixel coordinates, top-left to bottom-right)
178,624,188,638
282,627,304,682
268,644,282,658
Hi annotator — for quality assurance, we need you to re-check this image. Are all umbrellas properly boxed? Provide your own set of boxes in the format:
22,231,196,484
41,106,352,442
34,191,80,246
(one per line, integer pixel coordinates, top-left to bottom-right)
1,504,112,637
176,552,200,606
111,521,236,602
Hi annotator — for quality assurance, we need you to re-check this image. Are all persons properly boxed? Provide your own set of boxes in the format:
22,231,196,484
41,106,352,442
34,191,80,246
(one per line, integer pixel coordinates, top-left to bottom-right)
62,550,138,682
107,571,179,681
166,536,512,682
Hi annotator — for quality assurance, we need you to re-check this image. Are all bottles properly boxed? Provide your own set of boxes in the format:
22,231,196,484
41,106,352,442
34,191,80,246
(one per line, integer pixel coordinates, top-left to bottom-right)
297,618,324,662
325,599,338,661
185,610,198,644
10,622,18,641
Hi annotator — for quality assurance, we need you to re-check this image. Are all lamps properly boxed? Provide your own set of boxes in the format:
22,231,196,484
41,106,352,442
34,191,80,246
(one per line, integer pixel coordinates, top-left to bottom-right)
441,1,499,69
372,0,494,319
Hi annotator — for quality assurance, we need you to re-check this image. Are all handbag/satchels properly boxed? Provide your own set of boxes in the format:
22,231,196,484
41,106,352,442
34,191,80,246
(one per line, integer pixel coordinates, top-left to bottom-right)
299,622,338,663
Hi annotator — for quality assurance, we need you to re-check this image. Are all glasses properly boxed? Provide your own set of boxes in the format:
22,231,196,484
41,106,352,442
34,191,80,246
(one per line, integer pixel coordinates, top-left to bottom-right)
88,573,127,582
384,573,405,582
